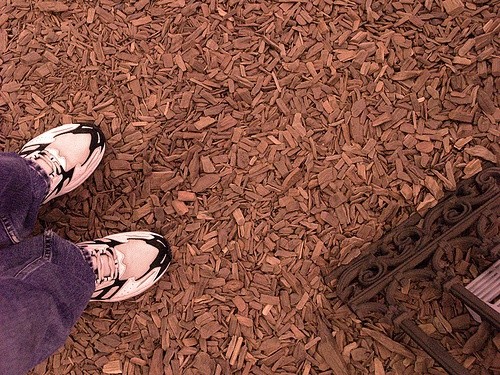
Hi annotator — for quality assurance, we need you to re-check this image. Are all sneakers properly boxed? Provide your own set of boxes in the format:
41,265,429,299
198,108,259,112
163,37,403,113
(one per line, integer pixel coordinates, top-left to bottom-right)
19,121,108,204
70,229,173,303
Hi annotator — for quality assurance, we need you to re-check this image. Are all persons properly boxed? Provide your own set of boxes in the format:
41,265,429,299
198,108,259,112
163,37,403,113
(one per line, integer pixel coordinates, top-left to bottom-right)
1,121,172,375
